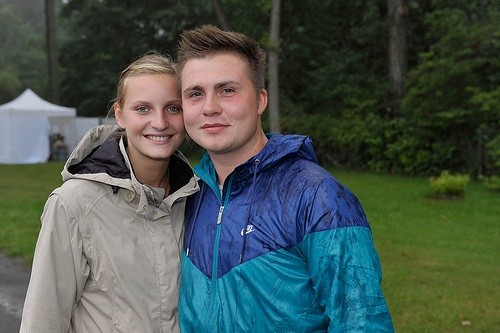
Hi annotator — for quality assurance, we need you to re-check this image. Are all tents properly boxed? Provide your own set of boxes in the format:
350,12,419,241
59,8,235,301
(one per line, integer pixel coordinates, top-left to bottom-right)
1,88,78,166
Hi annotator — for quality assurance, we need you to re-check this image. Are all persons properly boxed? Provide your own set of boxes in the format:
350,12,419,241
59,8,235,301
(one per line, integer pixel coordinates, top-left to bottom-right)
174,22,396,333
14,48,202,333
48,133,67,162
